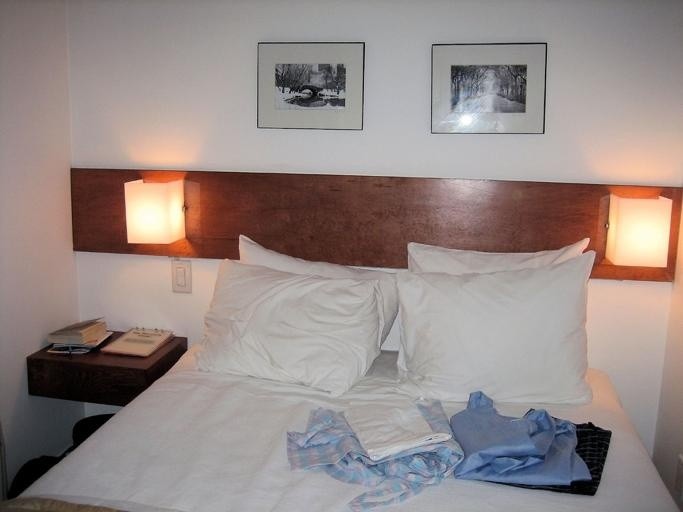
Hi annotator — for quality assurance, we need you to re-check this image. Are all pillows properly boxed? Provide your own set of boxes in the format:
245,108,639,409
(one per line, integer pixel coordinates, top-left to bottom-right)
185,259,398,397
395,271,593,404
407,237,591,272
238,235,399,345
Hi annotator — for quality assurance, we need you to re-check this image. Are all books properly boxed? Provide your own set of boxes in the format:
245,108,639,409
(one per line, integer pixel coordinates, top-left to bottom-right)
46,316,114,354
100,327,176,358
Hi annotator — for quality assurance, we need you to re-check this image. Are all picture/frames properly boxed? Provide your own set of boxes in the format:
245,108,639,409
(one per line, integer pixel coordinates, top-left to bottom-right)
427,39,550,138
254,39,367,136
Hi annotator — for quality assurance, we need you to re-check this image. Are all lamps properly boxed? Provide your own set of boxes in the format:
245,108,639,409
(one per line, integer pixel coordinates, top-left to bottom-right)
121,171,186,247
600,186,675,271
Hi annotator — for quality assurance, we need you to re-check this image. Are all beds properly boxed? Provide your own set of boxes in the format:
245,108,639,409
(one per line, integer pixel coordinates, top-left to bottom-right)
2,349,682,512
